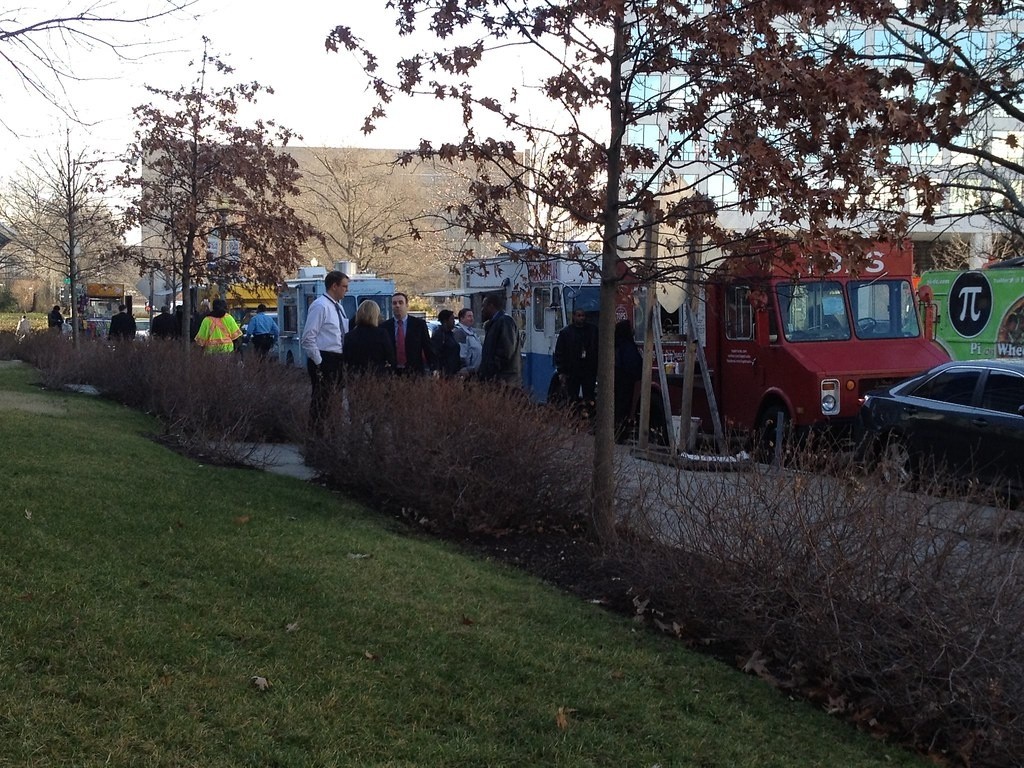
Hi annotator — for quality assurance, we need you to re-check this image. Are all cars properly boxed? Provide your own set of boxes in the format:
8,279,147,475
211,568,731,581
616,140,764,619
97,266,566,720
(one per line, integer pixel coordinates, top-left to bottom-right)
855,359,1024,497
135,321,151,337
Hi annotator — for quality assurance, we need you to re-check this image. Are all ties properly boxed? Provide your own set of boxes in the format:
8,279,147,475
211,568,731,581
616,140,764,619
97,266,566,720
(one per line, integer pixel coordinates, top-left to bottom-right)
323,294,345,349
396,320,407,365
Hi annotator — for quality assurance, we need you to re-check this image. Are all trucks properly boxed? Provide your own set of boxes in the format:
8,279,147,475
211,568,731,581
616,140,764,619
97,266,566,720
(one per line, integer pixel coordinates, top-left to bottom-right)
418,242,602,410
187,282,280,333
276,259,397,371
63,283,124,341
901,267,1024,361
611,227,954,467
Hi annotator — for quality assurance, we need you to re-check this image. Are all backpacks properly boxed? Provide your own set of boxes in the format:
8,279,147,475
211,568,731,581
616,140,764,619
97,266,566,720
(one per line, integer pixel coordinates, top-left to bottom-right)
457,323,482,373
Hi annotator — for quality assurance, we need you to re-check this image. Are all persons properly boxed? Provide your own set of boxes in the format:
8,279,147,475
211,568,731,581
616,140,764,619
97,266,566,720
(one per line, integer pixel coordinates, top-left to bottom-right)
108,305,136,342
194,299,243,354
344,298,394,367
15,315,30,337
613,320,643,444
378,293,439,377
430,310,461,374
48,306,64,331
103,303,112,317
451,308,482,369
245,304,280,355
553,308,598,435
152,303,211,342
477,294,521,389
301,271,351,466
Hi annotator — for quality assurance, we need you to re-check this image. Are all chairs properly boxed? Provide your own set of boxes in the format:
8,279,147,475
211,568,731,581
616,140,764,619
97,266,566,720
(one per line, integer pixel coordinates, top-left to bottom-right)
808,303,842,330
